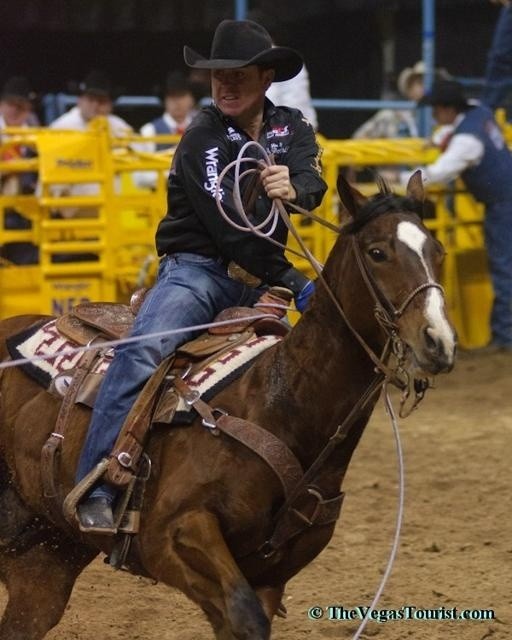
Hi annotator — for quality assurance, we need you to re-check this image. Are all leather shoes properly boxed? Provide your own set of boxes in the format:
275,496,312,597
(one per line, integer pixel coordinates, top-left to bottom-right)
74,497,116,533
472,341,511,356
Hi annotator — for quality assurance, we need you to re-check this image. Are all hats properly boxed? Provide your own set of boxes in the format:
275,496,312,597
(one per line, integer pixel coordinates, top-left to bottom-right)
417,80,477,111
398,60,424,97
184,20,303,82
67,69,126,100
154,73,207,102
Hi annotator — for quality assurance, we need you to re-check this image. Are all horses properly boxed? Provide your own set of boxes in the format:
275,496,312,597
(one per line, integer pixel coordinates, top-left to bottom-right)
0,168,458,640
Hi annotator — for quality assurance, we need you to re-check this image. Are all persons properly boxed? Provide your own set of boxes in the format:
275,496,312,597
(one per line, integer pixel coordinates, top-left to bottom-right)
41,75,155,262
0,79,100,265
64,19,332,536
347,63,463,216
378,79,511,359
253,33,318,137
140,76,211,187
